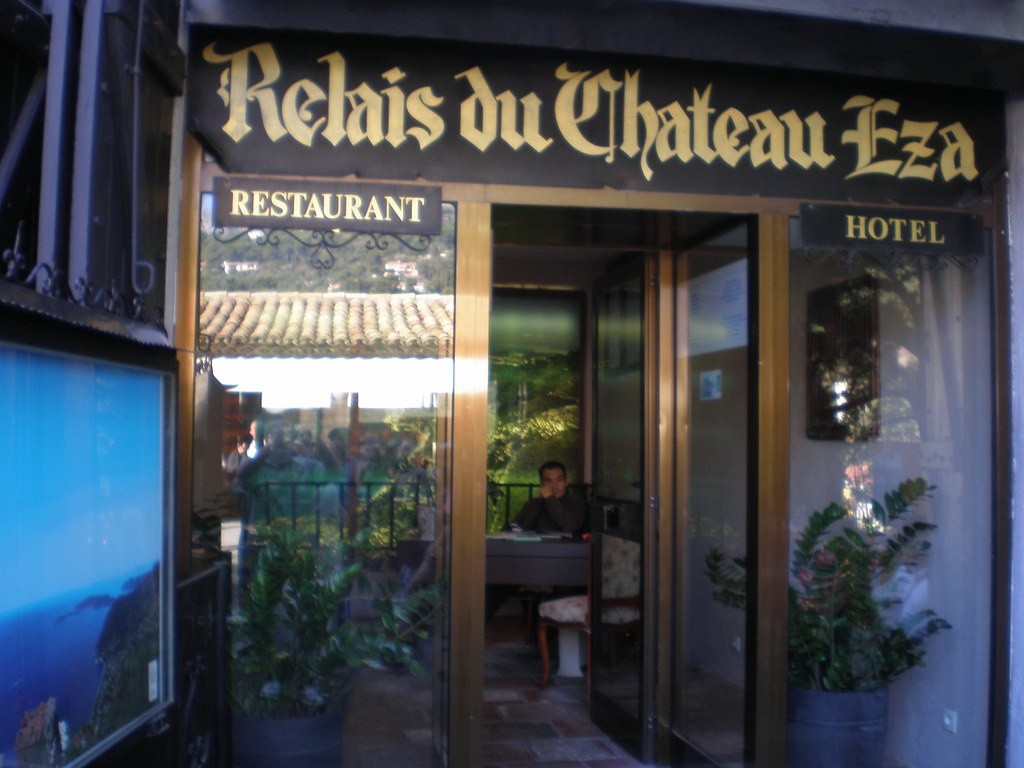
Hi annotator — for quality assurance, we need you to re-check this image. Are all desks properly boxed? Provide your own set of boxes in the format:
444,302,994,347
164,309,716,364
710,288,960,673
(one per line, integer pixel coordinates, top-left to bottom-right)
477,530,588,641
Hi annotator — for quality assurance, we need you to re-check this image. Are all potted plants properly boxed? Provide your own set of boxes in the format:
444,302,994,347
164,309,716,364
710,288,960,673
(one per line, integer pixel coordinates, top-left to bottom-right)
705,477,953,767
215,518,443,767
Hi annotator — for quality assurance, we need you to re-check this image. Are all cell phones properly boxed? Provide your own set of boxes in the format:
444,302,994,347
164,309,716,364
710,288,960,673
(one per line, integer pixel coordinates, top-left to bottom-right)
509,523,521,532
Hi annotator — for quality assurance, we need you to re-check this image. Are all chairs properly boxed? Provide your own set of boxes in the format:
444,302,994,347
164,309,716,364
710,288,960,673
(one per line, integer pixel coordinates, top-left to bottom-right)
539,532,643,690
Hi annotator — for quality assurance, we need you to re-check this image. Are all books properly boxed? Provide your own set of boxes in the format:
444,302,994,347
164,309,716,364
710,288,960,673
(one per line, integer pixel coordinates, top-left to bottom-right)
513,533,541,541
542,534,561,538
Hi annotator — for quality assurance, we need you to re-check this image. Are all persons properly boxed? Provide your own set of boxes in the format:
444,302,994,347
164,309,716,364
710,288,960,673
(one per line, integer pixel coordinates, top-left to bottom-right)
485,461,585,626
225,421,437,704
